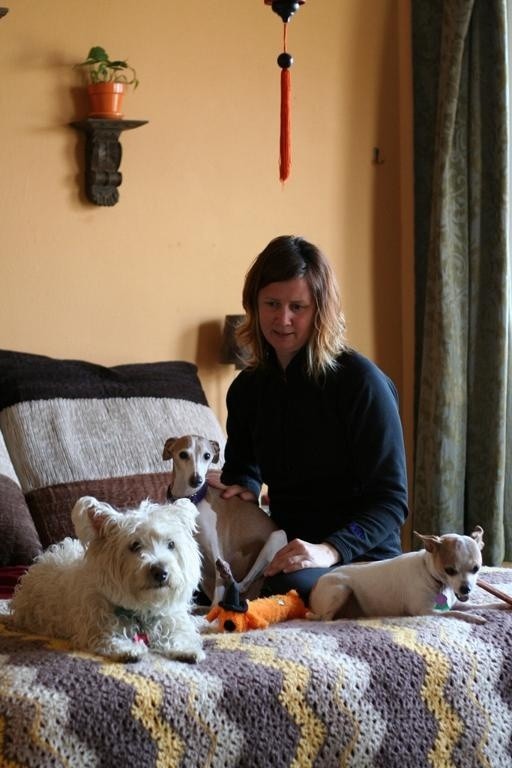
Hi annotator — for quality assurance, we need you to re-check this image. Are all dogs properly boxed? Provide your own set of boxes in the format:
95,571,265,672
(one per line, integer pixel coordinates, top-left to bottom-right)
11,495,206,663
304,525,485,621
162,434,289,611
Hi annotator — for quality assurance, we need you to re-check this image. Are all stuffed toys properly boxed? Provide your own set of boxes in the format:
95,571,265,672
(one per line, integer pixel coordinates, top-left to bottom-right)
205,557,308,632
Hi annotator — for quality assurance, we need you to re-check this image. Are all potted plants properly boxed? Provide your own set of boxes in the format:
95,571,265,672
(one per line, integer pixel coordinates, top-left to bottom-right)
72,45,139,118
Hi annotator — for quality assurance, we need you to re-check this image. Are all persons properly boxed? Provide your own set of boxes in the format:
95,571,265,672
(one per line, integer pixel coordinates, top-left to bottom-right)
207,236,410,609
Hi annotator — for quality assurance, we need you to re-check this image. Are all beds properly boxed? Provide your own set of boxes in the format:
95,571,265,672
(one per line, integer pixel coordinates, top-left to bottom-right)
2,562,510,765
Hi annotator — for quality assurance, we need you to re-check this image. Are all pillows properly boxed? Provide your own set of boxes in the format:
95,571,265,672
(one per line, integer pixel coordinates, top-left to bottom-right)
0,430,51,590
2,347,229,553
